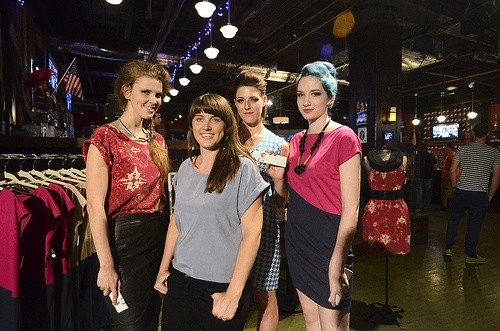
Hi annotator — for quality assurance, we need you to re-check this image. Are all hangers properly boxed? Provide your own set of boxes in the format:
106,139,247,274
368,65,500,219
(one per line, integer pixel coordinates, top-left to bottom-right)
0,154,87,190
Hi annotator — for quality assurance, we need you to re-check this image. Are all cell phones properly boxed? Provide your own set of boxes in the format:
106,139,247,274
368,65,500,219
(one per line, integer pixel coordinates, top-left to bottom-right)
107,291,128,313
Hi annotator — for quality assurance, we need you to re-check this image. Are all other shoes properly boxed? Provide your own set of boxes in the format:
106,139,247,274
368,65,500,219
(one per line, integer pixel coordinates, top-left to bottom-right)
442,248,452,256
465,254,488,264
423,211,431,215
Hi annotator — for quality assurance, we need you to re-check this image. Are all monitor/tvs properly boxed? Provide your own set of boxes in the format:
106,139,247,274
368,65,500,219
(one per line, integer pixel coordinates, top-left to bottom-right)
429,122,460,142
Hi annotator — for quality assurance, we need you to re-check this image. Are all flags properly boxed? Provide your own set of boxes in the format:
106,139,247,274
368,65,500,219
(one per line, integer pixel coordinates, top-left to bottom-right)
64,60,84,100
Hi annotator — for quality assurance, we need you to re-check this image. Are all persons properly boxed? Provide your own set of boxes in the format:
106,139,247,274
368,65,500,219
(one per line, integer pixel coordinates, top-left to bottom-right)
414,143,437,214
443,122,500,264
439,144,460,210
154,92,271,331
285,61,361,331
227,70,290,331
362,150,410,255
82,59,171,331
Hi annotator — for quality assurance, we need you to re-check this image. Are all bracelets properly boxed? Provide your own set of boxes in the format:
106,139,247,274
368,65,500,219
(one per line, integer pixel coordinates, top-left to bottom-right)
451,187,456,188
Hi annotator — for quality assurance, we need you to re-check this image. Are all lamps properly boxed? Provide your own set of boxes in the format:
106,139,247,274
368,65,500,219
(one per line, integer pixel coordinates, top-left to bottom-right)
273,95,289,124
163,0,239,103
466,92,478,119
437,96,447,123
411,96,421,125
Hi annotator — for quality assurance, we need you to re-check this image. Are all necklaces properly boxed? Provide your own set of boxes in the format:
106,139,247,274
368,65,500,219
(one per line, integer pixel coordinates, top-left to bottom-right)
118,116,150,141
295,116,331,176
